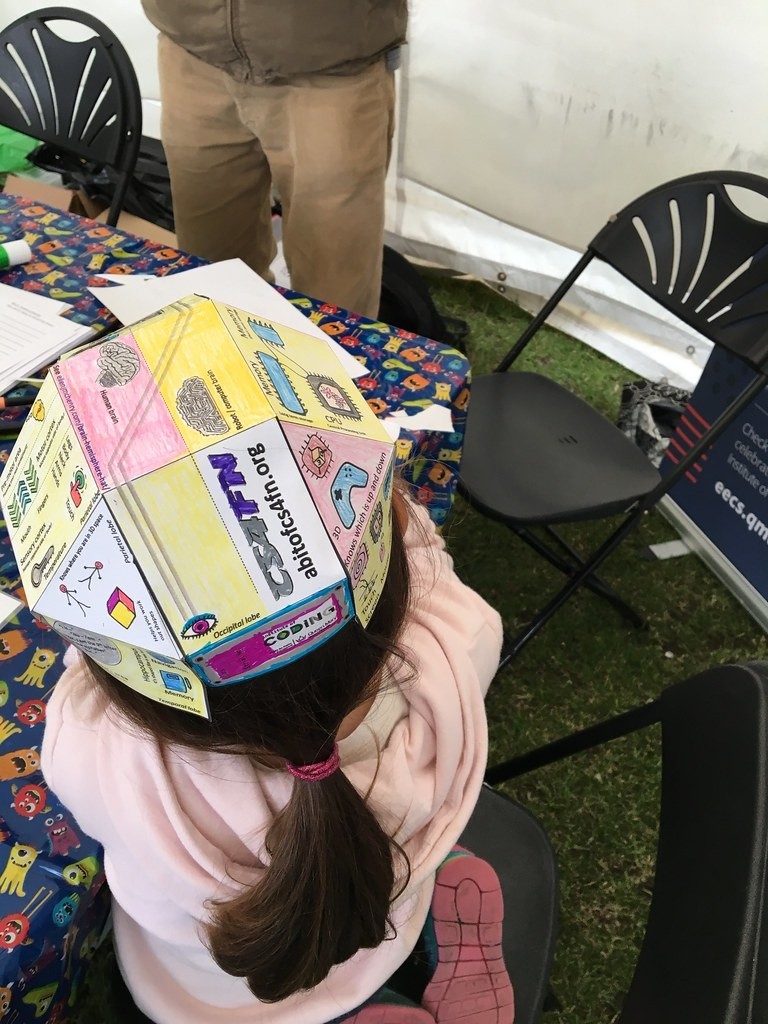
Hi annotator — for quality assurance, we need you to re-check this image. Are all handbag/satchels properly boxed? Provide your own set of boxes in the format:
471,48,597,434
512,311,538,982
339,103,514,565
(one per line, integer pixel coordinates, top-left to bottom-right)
0,124,45,172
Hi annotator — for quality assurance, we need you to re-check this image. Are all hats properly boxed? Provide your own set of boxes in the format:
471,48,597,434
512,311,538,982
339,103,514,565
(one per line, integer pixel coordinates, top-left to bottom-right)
0,292,396,726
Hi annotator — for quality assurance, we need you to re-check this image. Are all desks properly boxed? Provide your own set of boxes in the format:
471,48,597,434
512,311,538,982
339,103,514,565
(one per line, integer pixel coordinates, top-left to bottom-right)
0,195,470,1024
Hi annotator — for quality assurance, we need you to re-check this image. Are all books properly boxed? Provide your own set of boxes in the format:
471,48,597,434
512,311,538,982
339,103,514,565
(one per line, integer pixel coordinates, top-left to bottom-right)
1,298,98,393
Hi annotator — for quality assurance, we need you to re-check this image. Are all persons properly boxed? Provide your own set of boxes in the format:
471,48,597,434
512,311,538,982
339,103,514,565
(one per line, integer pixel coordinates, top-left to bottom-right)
0,294,516,1024
139,0,408,323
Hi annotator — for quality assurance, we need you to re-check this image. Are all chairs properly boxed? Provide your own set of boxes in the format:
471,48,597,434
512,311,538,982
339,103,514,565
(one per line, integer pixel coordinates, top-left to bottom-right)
0,7,142,230
445,170,768,667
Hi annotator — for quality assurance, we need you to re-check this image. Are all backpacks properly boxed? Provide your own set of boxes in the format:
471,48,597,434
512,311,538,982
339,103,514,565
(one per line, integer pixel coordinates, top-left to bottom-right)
378,243,470,355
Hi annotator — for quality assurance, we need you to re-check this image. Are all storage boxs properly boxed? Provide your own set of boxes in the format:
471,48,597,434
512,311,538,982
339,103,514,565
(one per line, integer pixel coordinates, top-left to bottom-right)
92,209,179,251
5,174,95,223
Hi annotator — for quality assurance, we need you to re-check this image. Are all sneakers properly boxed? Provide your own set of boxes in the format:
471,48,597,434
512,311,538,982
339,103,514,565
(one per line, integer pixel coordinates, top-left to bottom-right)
416,843,515,1024
326,987,435,1024
